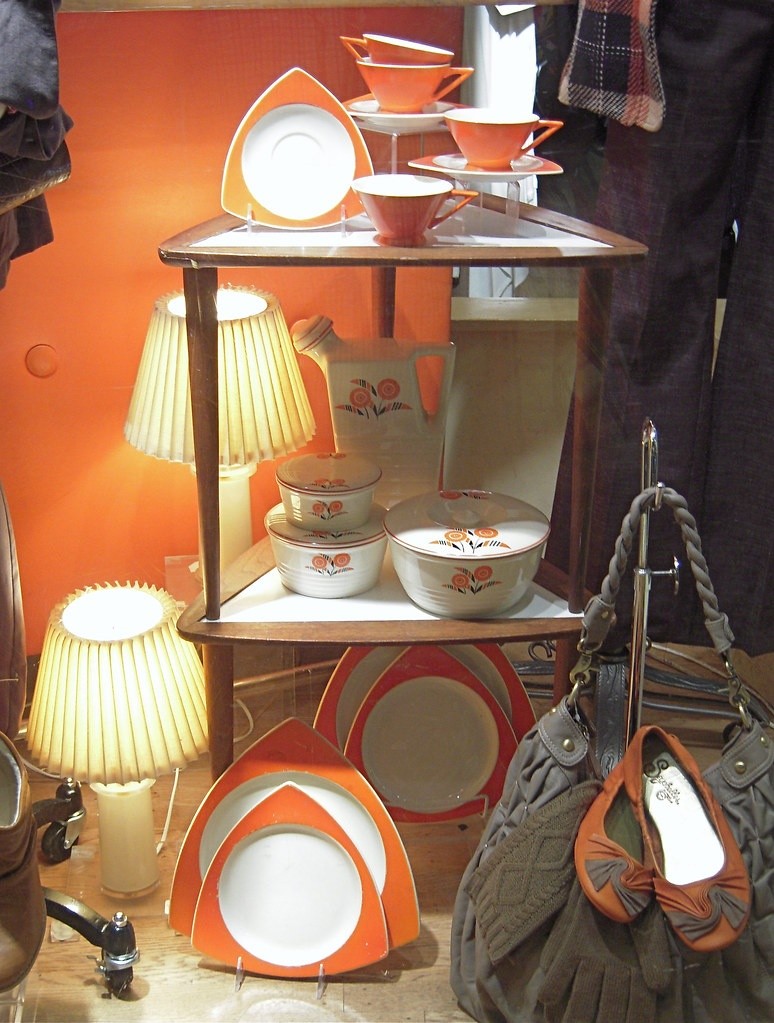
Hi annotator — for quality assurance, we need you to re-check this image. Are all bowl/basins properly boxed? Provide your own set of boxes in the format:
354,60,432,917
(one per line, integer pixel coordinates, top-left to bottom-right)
264,501,394,599
275,450,382,531
382,493,554,619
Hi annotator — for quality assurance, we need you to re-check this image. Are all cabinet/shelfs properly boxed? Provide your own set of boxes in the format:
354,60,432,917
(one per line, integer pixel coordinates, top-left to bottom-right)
160,89,656,826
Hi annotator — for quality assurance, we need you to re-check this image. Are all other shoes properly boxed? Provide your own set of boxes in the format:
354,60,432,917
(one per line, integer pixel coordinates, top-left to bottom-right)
574,725,752,954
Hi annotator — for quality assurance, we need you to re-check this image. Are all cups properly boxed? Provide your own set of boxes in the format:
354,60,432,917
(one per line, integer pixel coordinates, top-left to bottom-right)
444,107,563,171
289,311,456,512
340,33,475,112
351,173,478,246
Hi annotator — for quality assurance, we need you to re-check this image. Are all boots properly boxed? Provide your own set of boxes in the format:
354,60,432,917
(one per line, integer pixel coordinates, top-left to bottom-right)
1,730,48,998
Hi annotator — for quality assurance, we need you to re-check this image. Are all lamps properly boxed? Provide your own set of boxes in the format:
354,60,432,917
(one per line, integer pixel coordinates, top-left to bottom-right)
23,580,211,902
125,287,317,587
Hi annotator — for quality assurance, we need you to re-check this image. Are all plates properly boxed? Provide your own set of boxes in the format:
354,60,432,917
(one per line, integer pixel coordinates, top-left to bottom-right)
221,67,380,232
167,715,421,956
406,153,564,182
189,780,388,978
313,640,539,758
342,645,521,822
336,92,476,131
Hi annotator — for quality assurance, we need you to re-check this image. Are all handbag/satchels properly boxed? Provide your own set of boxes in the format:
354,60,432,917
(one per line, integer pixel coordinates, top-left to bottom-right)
448,490,774,1023
